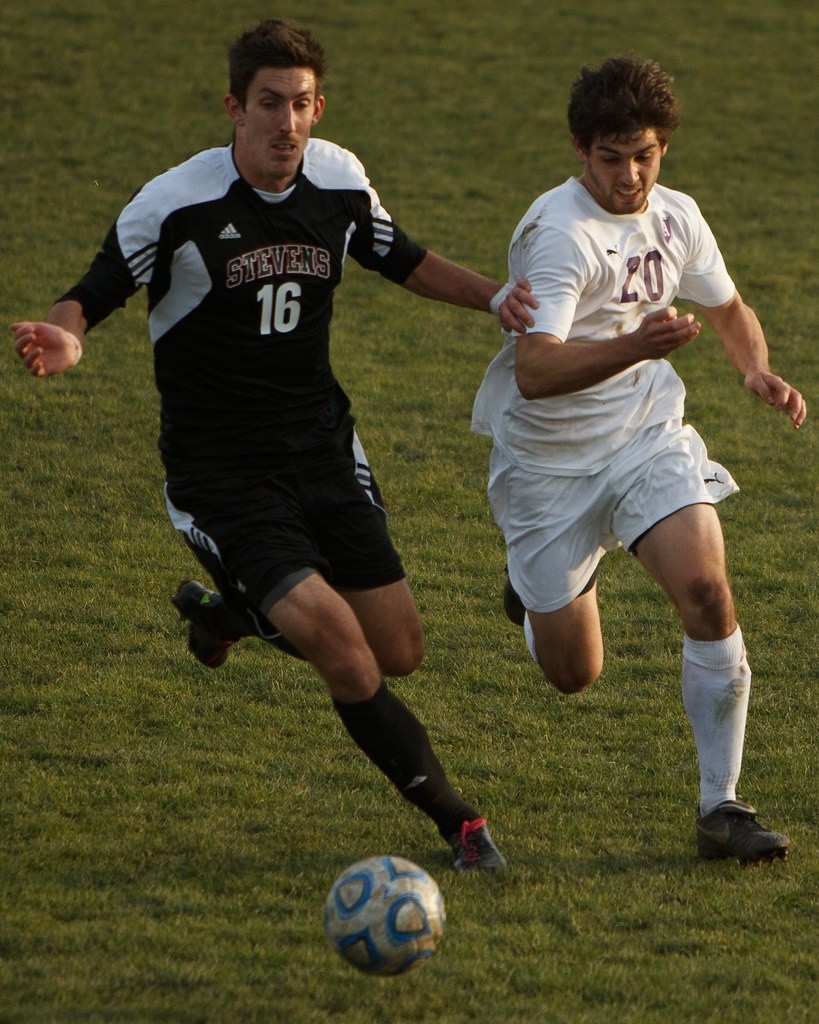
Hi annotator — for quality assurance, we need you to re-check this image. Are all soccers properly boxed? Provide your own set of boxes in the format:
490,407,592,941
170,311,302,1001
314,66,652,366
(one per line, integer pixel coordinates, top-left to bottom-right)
322,854,446,976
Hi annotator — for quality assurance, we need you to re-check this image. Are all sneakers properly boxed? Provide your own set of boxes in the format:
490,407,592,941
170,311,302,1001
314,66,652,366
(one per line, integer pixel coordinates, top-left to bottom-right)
171,578,240,668
695,800,789,863
502,564,526,627
441,818,506,874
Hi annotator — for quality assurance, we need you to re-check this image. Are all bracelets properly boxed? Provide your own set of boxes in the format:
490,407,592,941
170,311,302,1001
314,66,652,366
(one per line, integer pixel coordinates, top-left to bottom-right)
490,283,516,315
64,331,83,367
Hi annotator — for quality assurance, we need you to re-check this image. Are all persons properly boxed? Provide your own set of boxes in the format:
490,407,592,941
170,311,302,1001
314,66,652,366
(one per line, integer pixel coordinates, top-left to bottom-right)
12,15,541,881
469,54,810,860
